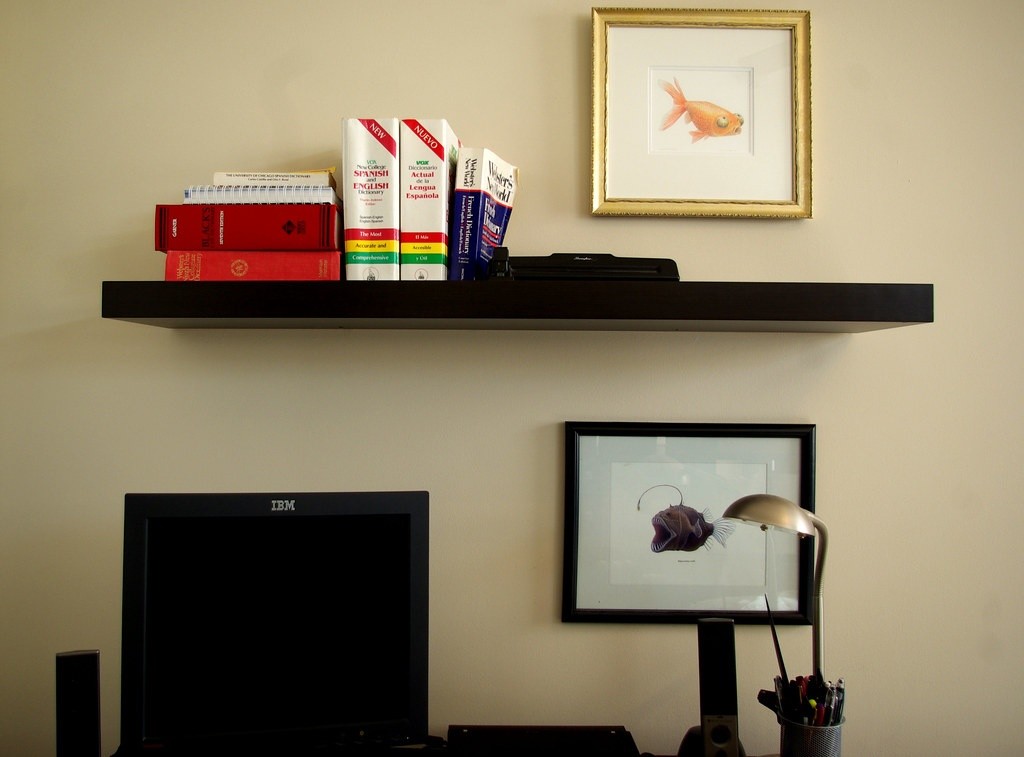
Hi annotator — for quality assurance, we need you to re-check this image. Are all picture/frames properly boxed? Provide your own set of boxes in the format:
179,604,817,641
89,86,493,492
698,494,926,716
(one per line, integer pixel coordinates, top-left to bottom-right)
562,419,817,627
590,5,815,220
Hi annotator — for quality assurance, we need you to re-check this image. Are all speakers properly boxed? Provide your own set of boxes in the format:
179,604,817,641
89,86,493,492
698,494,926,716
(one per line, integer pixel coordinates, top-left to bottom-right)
698,619,741,754
55,648,101,756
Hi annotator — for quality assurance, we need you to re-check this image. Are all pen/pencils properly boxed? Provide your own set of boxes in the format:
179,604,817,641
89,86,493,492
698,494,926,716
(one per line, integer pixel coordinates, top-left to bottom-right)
755,667,844,757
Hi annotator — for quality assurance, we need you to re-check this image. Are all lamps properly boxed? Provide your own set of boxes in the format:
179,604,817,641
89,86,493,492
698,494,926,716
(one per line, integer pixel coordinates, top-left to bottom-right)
718,491,828,680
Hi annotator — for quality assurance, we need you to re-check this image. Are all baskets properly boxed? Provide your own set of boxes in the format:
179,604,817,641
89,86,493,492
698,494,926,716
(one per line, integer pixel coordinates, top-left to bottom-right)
775,710,846,757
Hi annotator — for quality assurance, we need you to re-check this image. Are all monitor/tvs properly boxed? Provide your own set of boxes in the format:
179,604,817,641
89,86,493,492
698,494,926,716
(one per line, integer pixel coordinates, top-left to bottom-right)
120,492,430,757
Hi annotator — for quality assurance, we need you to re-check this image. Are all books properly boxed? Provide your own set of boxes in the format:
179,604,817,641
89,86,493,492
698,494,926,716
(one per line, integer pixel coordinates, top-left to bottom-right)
345,116,521,280
154,169,341,280
